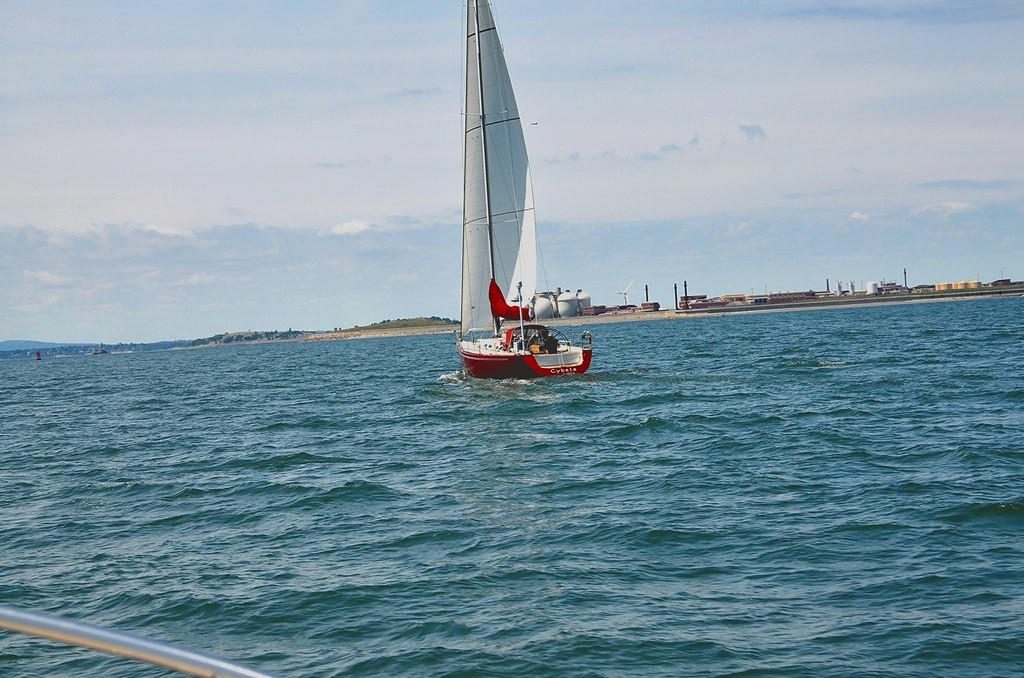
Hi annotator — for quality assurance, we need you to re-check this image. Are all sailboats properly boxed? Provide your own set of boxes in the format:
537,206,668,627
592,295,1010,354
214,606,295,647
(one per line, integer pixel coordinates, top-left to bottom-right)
454,0,593,379
